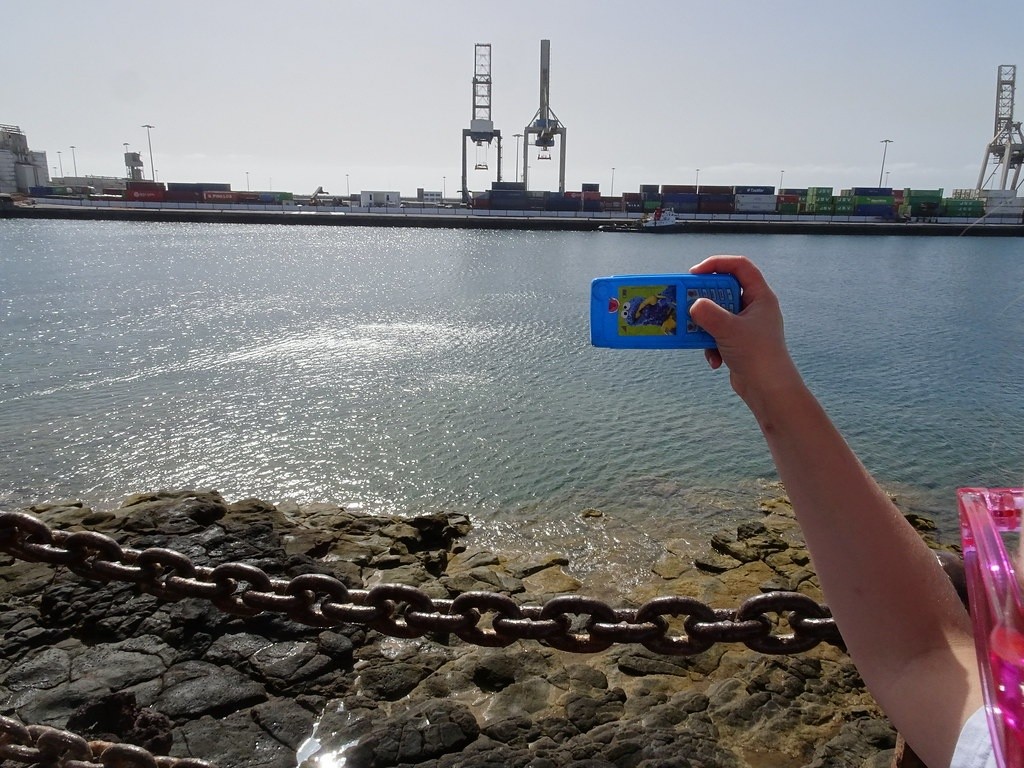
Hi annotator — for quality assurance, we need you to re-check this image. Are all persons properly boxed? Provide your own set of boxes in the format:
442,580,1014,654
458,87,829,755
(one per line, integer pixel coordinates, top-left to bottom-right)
653,207,666,221
690,255,1024,768
641,212,650,224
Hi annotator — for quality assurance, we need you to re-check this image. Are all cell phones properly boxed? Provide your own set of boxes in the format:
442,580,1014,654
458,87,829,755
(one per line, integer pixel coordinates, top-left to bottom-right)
589,274,740,349
